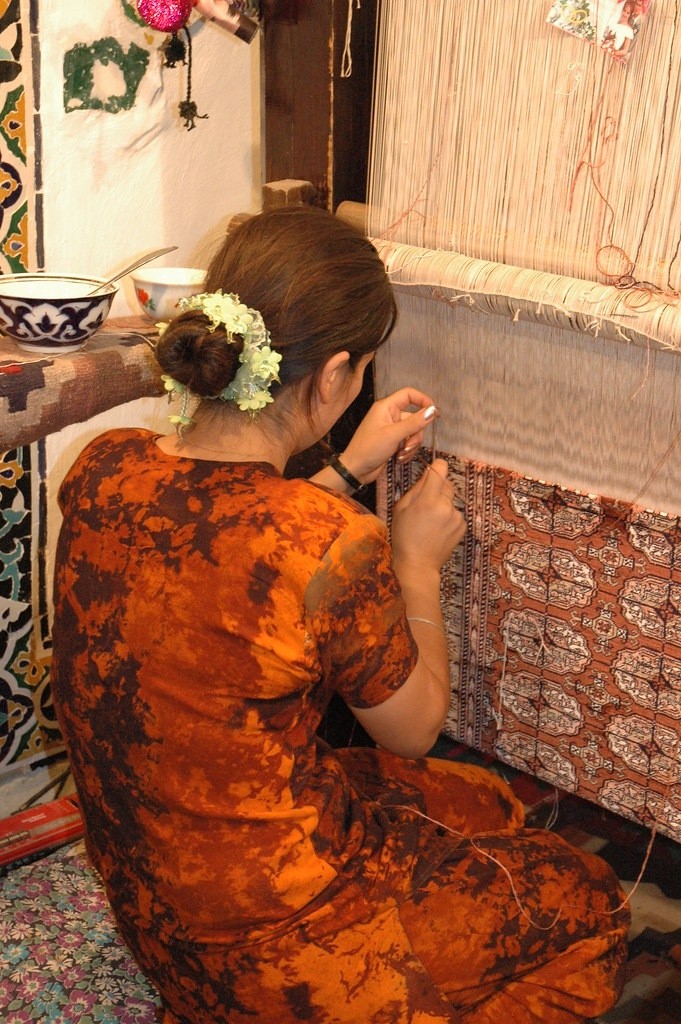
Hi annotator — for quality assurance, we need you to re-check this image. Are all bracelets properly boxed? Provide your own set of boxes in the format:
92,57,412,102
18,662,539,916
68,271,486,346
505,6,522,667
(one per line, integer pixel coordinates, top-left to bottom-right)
329,456,367,493
407,617,445,633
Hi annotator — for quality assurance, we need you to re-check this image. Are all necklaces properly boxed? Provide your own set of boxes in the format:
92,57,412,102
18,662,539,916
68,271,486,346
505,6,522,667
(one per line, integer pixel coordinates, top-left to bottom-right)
184,439,284,471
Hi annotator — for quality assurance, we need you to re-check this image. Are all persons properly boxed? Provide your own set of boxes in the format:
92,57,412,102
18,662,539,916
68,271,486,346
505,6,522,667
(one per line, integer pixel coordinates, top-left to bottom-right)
51,206,633,1024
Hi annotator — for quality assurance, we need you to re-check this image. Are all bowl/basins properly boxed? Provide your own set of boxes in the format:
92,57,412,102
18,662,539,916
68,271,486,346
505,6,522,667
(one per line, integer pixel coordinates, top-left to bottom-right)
129,266,210,320
0,273,120,353
